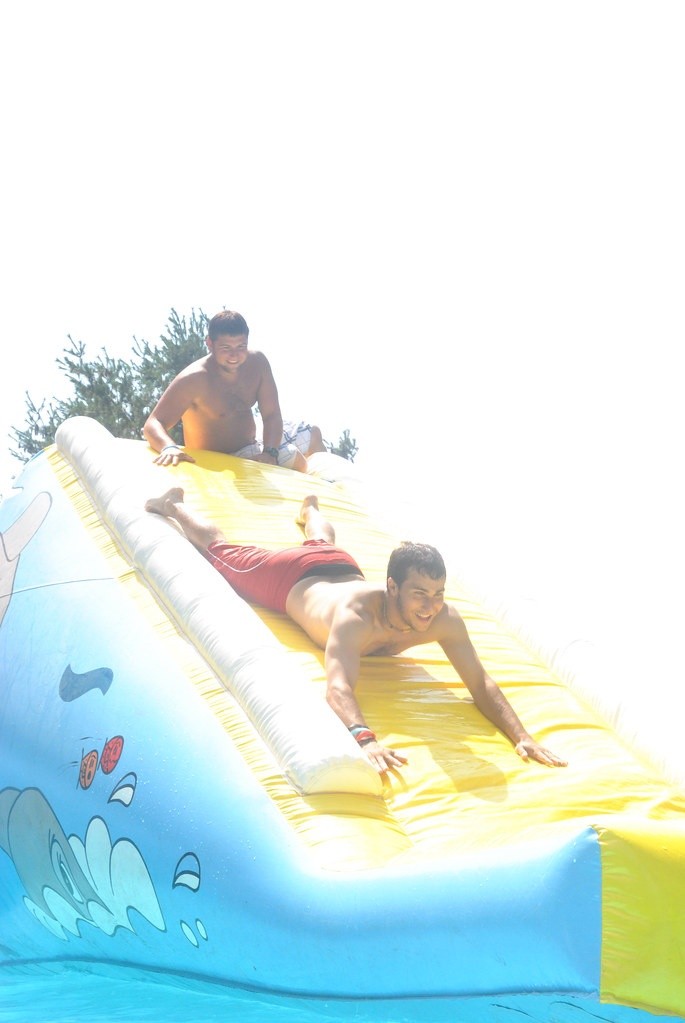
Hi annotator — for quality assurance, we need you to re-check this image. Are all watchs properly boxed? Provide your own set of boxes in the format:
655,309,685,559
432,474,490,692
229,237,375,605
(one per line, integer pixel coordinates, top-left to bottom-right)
263,448,279,457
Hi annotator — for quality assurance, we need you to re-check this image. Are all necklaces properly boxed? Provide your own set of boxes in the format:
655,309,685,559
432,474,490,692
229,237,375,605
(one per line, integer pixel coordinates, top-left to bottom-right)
384,601,411,631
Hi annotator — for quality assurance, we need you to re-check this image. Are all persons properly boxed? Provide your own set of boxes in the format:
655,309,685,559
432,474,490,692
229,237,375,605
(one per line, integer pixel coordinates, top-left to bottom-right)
144,487,567,775
143,311,326,474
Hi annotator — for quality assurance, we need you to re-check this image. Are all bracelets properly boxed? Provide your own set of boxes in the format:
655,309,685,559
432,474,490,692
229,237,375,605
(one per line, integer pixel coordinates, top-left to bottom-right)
163,445,179,451
347,725,377,747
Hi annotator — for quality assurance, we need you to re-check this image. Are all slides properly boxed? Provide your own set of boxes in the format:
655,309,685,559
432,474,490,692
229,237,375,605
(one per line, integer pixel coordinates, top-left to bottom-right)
0,406,685,1011
